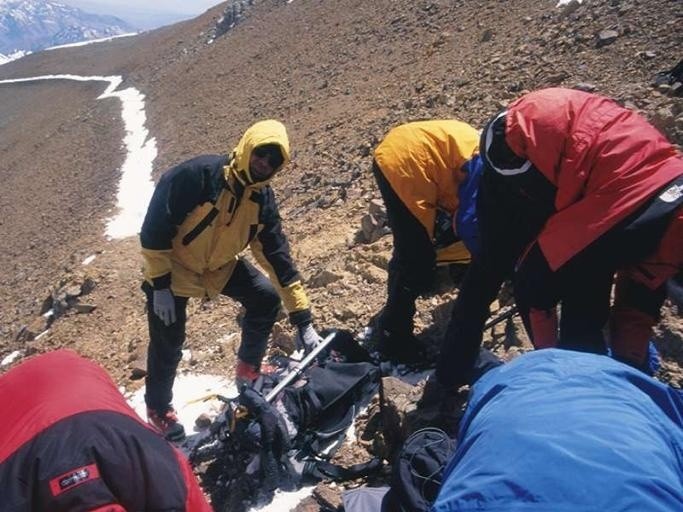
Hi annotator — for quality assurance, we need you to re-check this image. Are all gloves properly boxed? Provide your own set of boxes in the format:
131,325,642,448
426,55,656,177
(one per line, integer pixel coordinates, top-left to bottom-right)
295,321,326,359
152,288,177,328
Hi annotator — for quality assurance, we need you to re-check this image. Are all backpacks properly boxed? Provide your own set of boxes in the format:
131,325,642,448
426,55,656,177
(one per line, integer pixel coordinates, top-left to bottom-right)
272,326,382,457
180,383,291,509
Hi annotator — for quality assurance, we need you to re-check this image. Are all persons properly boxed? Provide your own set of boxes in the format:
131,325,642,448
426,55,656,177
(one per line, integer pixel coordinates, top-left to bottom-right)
0,350,215,512
420,88,683,408
139,120,324,443
369,118,487,366
430,344,683,511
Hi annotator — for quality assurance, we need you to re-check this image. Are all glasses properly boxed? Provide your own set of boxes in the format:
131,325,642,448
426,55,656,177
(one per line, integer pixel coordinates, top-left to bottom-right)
255,148,280,168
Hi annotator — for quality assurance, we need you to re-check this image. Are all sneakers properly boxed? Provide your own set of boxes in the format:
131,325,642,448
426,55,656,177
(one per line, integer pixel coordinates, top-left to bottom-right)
367,329,421,361
415,378,456,409
146,405,184,441
233,358,260,388
